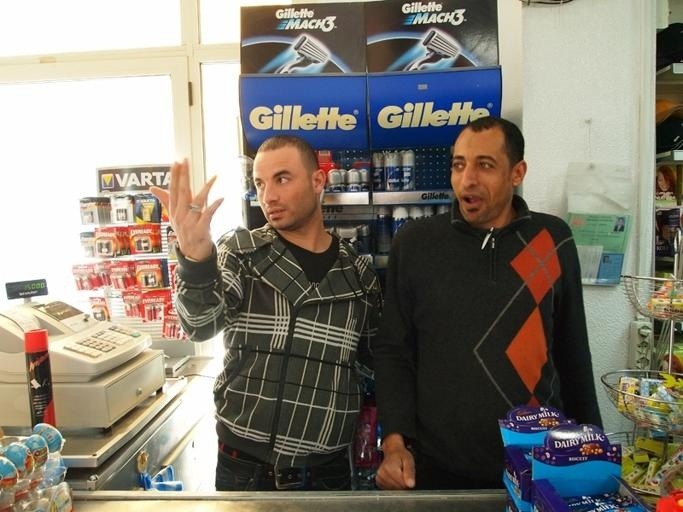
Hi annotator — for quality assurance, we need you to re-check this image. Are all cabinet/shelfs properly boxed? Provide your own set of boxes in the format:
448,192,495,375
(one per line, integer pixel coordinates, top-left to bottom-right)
237,66,502,269
656,61,682,290
62,377,203,491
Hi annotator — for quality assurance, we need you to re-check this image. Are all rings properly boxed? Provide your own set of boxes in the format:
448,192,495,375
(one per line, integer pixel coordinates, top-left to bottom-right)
188,202,201,212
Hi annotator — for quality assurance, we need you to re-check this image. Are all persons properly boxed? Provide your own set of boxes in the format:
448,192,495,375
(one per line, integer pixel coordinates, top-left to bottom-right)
656,165,675,197
149,135,384,492
613,218,625,232
371,120,605,490
